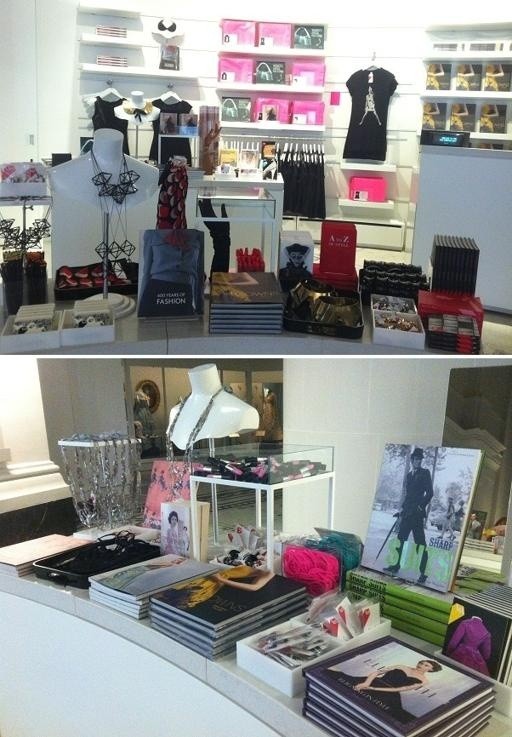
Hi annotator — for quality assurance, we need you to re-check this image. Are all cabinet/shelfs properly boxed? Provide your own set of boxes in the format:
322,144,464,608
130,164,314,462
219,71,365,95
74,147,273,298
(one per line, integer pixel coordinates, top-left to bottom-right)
411,22,512,315
74,0,327,161
336,157,398,210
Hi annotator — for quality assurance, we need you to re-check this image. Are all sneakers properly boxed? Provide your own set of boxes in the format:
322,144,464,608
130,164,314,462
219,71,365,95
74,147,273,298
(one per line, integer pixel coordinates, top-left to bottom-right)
418,576,427,581
383,564,400,572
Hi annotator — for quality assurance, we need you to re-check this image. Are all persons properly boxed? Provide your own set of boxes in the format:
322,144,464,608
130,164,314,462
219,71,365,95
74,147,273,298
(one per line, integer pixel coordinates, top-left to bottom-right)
482,517,508,542
278,242,313,291
466,513,481,540
377,447,482,583
134,389,150,434
45,127,157,279
163,510,189,558
423,64,504,133
164,361,260,450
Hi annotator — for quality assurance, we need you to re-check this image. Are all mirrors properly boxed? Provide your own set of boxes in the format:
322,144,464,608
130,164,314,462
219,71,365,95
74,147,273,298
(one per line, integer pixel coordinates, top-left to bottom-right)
441,364,512,577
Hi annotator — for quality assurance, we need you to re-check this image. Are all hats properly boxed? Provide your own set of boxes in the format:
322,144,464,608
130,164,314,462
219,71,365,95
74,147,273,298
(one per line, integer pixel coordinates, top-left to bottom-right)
410,448,424,459
286,244,308,255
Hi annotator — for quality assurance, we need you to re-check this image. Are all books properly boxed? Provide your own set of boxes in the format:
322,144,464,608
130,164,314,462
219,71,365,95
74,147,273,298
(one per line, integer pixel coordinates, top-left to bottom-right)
136,228,205,337
0,534,512,737
210,270,285,336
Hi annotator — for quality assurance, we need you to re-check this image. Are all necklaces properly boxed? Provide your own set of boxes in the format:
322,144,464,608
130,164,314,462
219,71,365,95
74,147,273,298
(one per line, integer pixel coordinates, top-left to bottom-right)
59,431,142,532
91,147,140,280
165,385,224,497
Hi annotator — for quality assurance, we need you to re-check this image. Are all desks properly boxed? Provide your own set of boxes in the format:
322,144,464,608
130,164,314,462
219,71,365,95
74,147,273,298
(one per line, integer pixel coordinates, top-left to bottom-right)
0,528,512,737
0,168,285,282
